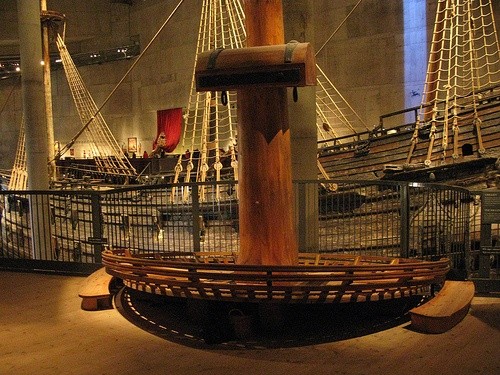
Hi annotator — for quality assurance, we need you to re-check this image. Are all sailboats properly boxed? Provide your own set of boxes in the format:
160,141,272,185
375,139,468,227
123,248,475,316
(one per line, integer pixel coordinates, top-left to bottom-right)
1,0,500,261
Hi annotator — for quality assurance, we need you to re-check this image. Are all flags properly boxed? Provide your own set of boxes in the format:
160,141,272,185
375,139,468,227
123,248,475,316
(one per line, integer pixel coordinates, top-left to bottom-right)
153,107,182,153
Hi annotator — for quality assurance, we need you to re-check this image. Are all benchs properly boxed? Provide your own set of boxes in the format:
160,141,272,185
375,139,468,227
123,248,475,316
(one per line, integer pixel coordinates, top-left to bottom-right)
409,279,476,335
78,262,133,311
